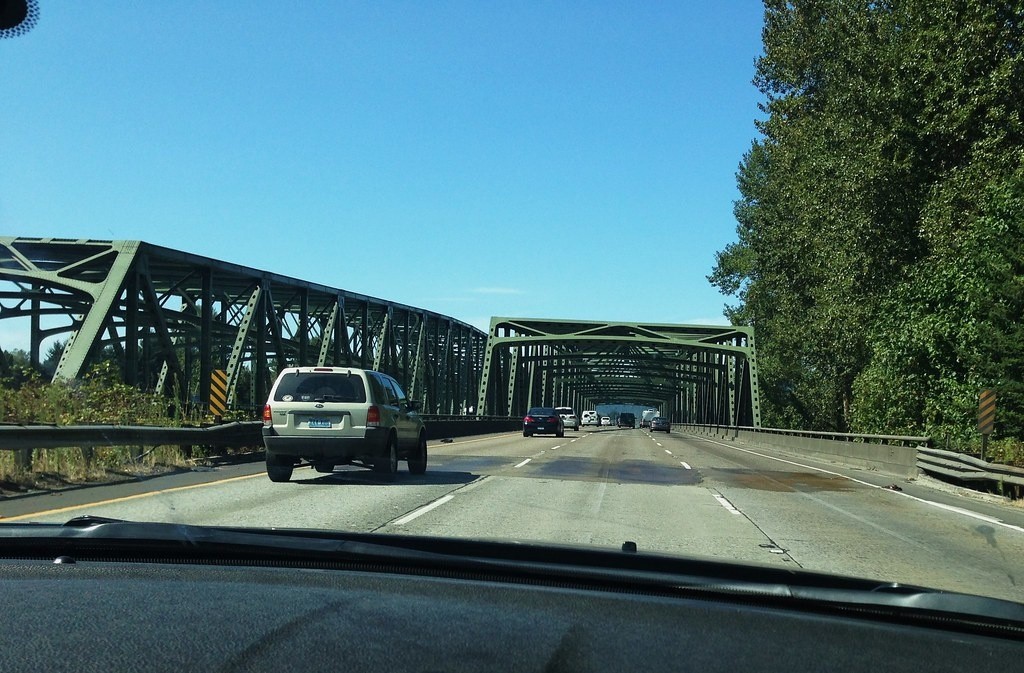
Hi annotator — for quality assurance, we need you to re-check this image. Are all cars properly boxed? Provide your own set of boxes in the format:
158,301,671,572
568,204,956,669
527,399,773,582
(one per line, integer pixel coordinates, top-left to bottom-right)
650,417,671,434
601,417,610,426
522,408,564,439
554,407,579,431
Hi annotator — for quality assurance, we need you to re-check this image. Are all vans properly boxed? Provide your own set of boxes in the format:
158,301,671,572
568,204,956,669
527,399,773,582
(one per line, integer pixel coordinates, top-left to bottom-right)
581,410,600,427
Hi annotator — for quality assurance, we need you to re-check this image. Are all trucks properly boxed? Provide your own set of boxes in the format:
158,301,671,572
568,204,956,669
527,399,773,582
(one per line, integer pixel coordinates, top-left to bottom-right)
640,408,660,429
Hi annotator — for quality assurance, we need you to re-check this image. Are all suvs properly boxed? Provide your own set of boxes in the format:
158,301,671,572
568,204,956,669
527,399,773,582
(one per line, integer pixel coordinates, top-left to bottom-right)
260,367,427,483
618,413,635,429
